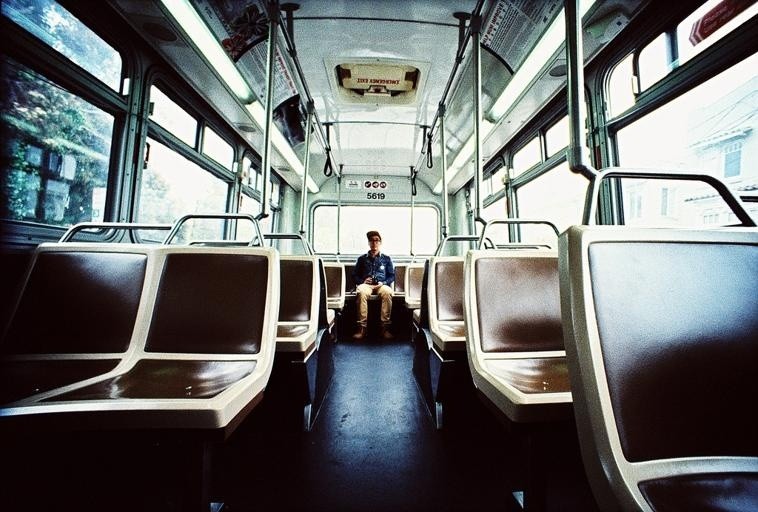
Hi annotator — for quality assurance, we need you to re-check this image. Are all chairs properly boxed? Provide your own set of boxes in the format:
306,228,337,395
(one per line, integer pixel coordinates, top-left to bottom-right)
404,254,434,344
314,253,346,344
246,234,330,354
557,164,758,512
466,219,574,431
414,239,466,424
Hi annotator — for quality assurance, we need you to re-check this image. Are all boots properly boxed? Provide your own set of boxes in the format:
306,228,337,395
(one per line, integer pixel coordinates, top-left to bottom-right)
380,324,393,341
352,326,366,339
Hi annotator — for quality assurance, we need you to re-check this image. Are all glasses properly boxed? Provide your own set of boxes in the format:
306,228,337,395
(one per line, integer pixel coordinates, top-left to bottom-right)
369,238,380,242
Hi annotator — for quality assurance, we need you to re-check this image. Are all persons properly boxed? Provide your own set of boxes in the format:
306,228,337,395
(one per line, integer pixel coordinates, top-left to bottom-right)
352,231,395,340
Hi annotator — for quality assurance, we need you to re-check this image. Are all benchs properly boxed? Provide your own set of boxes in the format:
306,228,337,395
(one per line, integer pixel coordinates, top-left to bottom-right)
346,264,405,299
0,215,280,431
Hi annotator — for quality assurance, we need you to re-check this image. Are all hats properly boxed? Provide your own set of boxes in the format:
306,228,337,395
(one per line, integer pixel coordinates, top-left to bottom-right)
366,231,380,240
